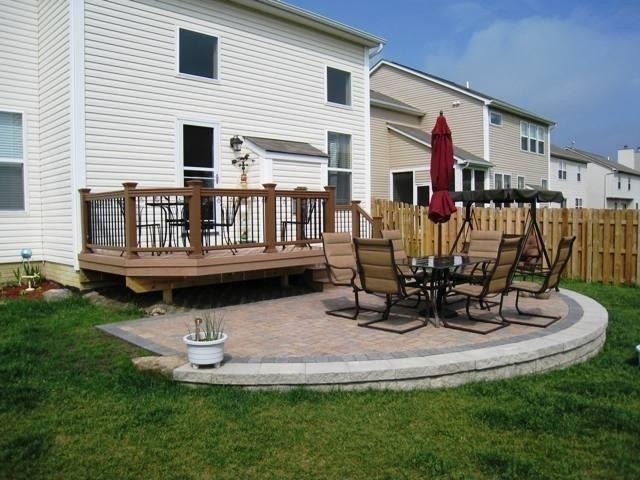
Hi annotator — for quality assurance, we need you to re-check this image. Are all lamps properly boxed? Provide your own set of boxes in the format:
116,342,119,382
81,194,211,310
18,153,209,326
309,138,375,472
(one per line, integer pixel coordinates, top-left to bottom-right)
230,135,243,151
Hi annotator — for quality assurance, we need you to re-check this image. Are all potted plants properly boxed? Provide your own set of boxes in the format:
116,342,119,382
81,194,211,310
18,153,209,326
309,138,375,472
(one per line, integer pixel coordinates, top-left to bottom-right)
182,305,228,370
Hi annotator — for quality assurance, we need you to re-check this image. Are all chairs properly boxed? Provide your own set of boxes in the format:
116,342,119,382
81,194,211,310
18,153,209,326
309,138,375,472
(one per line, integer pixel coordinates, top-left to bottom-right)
320,229,577,334
117,197,320,257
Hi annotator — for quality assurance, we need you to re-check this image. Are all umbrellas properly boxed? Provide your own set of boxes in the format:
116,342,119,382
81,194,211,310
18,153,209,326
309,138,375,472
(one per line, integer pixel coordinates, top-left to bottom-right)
422,109,459,310
